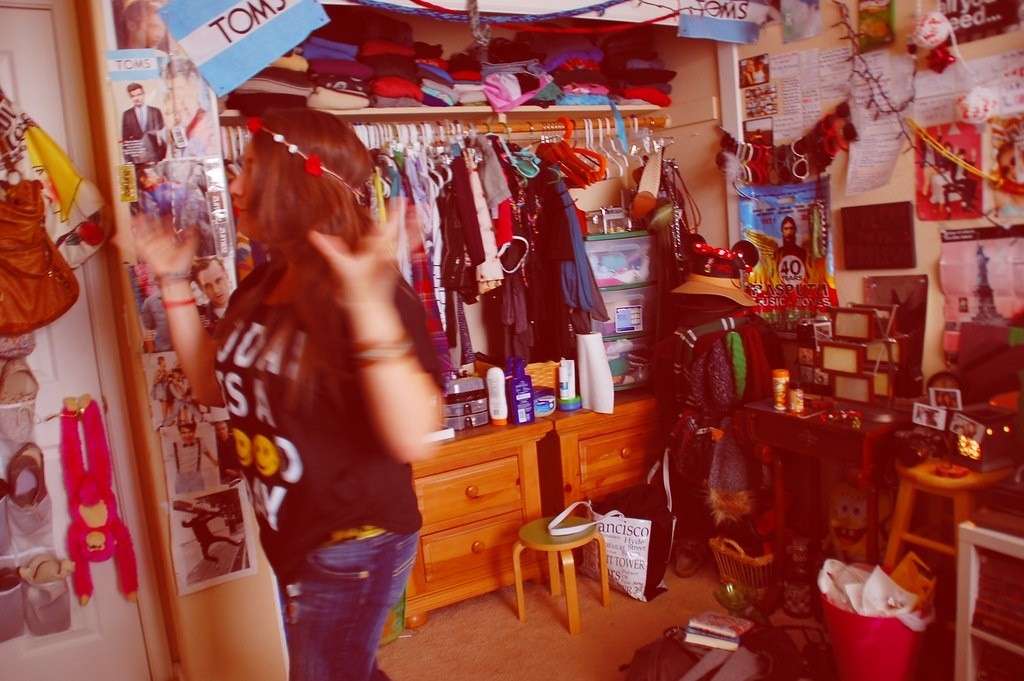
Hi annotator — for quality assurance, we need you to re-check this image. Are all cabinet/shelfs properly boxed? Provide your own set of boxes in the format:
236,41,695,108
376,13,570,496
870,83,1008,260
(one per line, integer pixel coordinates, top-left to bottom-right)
956,521,1023,681
402,417,553,629
537,394,672,518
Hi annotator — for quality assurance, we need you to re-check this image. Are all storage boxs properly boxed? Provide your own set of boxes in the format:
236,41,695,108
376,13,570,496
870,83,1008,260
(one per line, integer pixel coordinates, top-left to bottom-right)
708,538,777,596
584,232,660,391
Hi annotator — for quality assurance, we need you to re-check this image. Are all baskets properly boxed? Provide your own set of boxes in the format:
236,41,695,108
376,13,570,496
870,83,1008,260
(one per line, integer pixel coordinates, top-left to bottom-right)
708,536,775,599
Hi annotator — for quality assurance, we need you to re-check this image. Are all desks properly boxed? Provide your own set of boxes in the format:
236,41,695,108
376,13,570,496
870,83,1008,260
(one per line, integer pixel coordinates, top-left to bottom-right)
744,393,911,564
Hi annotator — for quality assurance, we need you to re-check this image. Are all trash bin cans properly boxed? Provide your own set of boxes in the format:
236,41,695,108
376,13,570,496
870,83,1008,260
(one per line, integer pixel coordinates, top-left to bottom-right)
817,550,942,681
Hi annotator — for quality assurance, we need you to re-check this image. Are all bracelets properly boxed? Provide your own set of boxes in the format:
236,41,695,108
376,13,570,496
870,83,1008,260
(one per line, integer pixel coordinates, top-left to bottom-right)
153,269,199,309
351,336,417,366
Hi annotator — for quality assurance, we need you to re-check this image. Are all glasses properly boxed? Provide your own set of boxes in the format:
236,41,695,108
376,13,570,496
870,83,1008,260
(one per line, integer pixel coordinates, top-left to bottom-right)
715,102,859,187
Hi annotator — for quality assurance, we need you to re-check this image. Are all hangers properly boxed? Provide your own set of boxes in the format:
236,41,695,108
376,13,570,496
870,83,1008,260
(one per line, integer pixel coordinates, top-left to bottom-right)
218,116,676,225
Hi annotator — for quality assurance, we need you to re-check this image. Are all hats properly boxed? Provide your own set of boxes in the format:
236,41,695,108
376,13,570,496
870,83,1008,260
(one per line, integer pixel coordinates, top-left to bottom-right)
670,258,758,308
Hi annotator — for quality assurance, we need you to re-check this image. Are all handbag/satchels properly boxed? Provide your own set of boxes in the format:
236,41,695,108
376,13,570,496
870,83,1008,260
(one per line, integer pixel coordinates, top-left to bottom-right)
0,181,79,335
0,92,113,269
580,483,677,602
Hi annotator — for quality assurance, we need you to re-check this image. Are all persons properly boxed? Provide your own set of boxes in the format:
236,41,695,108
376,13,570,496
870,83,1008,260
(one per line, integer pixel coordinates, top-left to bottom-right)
114,104,443,681
919,123,977,215
771,214,810,286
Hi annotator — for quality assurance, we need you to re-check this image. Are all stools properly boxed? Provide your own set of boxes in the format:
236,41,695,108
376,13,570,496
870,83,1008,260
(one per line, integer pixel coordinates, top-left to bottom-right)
882,455,1017,566
512,516,612,637
